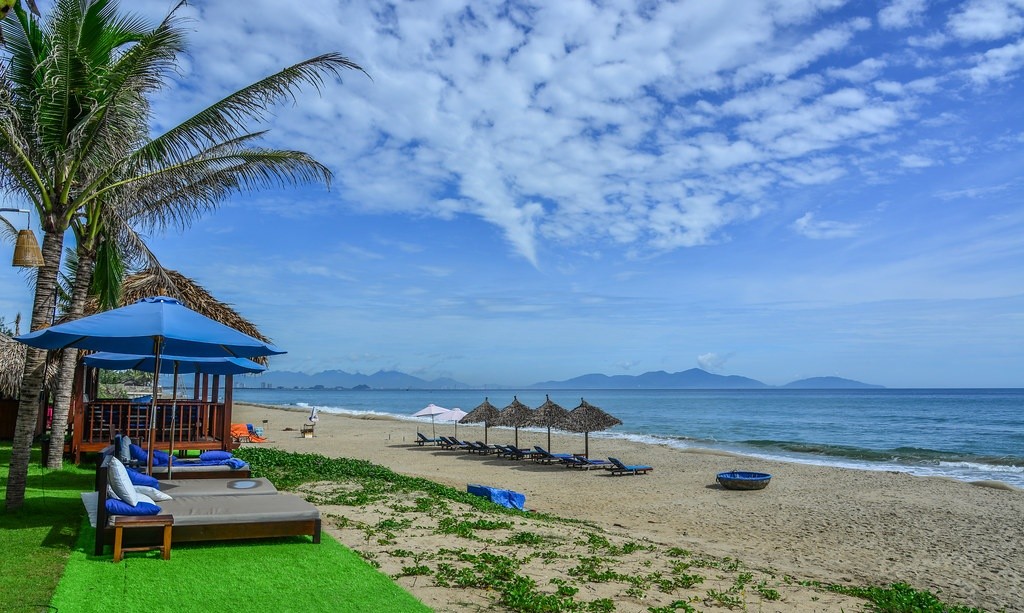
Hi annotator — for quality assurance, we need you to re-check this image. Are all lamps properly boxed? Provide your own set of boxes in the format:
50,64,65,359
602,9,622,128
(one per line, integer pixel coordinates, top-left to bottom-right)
0,207,47,267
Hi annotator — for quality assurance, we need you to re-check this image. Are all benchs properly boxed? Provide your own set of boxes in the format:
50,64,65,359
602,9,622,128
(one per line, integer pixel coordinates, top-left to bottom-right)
87,396,206,441
231,422,251,444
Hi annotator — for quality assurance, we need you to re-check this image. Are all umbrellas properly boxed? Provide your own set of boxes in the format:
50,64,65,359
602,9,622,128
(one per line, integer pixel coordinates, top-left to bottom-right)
553,396,623,462
458,396,503,454
413,403,449,447
50,268,277,421
82,350,270,482
515,394,571,465
9,287,290,491
433,407,472,449
489,394,537,462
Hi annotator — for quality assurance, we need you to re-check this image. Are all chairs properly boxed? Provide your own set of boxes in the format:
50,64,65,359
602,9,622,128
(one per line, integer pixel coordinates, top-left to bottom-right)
246,424,259,439
573,453,611,470
414,432,444,447
476,440,508,455
448,436,477,452
439,435,466,451
533,445,576,465
464,440,495,453
606,456,653,475
300,423,315,438
494,444,531,458
507,445,538,460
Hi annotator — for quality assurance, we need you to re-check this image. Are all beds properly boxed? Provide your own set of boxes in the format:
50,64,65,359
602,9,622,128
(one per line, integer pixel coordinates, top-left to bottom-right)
111,433,252,479
93,455,322,556
95,445,280,497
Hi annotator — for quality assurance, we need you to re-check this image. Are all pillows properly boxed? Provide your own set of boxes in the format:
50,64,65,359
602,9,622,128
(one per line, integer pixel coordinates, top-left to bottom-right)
105,498,162,517
120,435,133,461
133,484,174,503
107,456,139,507
199,450,234,461
125,465,161,490
130,444,160,466
106,483,157,508
152,449,180,464
